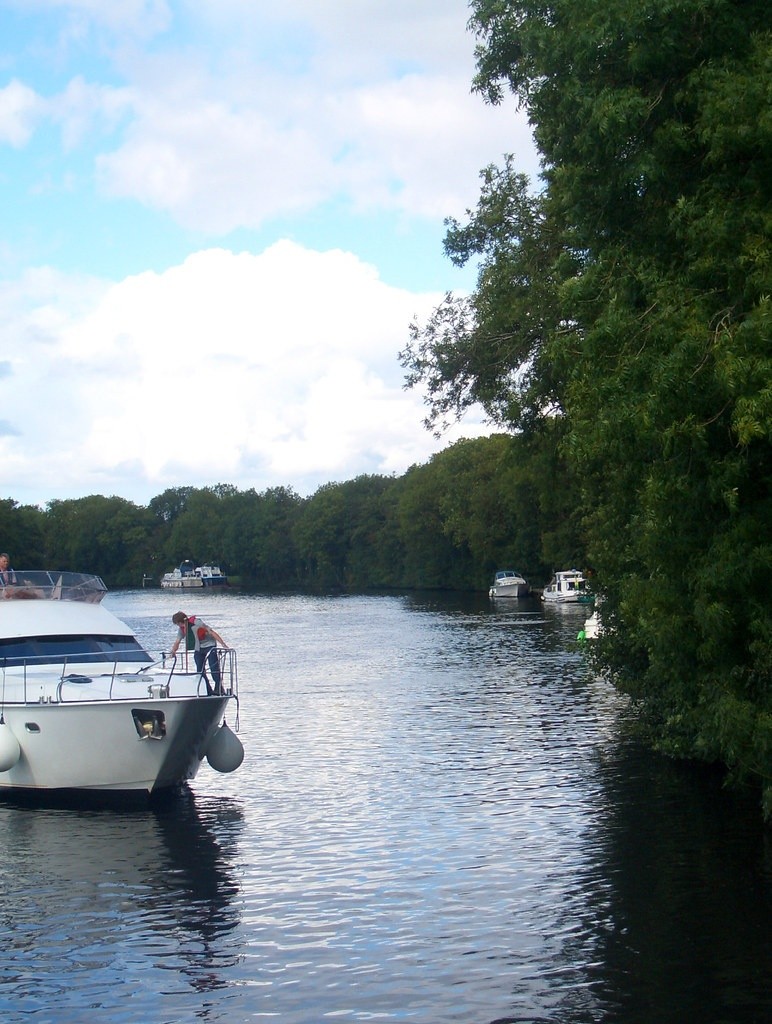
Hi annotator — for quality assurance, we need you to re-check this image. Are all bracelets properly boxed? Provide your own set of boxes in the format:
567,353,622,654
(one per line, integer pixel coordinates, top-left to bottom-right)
222,644,226,646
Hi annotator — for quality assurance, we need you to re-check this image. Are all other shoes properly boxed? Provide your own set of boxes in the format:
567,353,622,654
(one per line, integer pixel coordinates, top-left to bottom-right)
206,685,213,697
214,682,225,696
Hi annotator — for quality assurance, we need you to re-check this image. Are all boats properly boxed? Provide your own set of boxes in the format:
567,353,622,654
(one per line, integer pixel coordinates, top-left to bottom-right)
0,571,239,809
584,591,620,639
490,571,529,597
161,560,231,588
542,569,591,602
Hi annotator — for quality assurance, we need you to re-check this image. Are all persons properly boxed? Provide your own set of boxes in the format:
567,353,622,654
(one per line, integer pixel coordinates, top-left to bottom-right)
170,611,229,695
0,553,25,587
